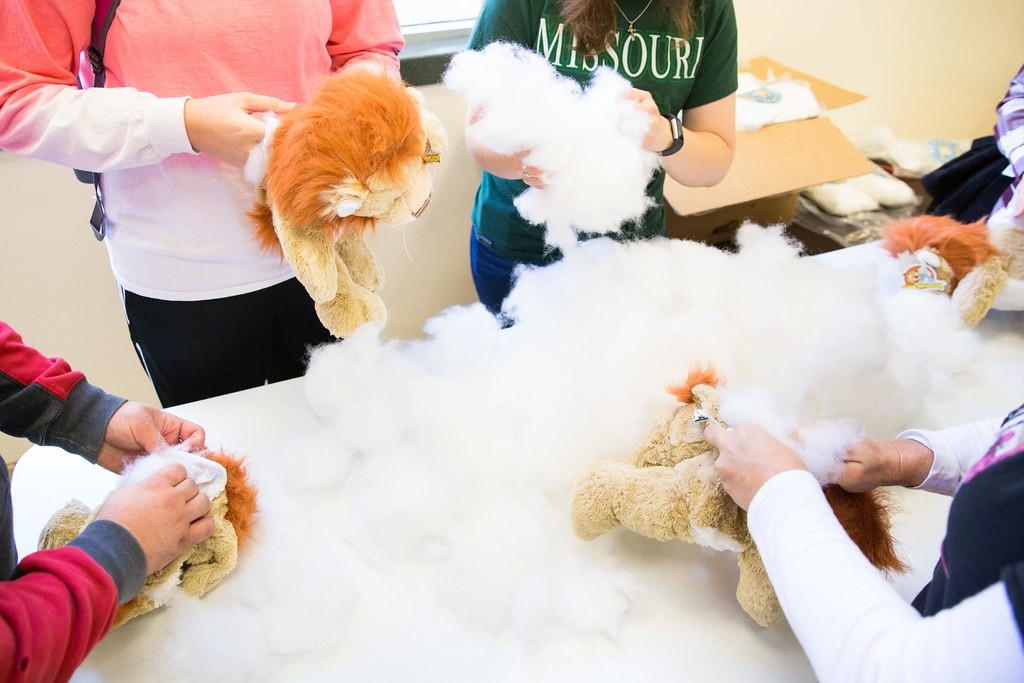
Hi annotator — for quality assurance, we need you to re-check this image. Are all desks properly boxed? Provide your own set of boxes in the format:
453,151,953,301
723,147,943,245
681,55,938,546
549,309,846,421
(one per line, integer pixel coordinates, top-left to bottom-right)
10,239,1024,683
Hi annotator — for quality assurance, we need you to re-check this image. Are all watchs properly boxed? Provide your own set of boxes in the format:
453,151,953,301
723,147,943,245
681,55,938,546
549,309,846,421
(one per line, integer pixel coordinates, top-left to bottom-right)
650,112,686,161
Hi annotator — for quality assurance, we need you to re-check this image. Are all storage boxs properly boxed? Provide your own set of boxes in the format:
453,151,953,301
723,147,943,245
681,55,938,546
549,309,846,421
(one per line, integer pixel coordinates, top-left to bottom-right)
663,54,875,247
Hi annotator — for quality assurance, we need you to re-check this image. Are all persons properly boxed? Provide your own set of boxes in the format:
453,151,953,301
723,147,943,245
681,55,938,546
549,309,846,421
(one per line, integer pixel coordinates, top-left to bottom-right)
702,401,1024,683
0,319,212,683
463,0,740,312
0,1,408,414
922,62,1024,228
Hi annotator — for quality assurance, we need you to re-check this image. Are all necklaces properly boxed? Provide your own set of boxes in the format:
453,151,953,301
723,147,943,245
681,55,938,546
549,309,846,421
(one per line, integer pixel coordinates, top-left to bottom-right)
614,1,652,41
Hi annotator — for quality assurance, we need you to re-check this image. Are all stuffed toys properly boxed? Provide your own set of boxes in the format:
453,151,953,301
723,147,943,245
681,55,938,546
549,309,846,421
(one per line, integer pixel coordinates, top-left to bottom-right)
36,433,259,633
572,360,911,630
235,64,445,342
881,212,1023,329
